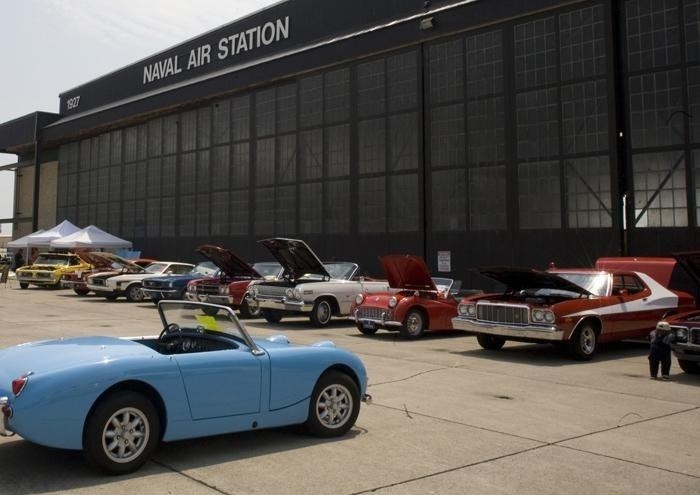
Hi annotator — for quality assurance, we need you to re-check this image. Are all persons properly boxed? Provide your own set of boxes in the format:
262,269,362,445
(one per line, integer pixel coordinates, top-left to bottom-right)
31,247,40,263
14,248,23,272
648,321,676,382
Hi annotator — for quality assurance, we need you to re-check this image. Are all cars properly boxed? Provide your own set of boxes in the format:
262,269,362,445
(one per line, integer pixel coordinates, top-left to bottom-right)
17,253,93,291
247,236,390,328
352,255,462,335
61,244,285,318
451,261,700,376
0,298,369,472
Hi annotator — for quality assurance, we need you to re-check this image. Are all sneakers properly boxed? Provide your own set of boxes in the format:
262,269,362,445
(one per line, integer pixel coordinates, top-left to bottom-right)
650,375,672,381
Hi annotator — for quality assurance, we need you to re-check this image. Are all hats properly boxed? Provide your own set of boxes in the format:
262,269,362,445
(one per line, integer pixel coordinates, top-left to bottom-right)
656,321,671,331
